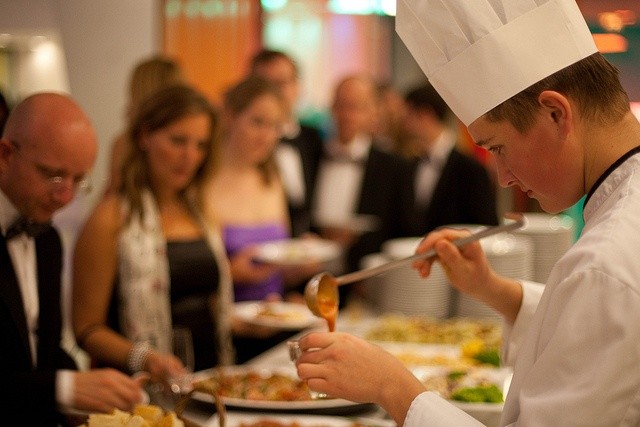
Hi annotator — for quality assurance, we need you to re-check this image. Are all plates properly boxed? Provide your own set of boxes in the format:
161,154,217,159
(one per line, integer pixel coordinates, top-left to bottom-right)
363,254,385,311
249,237,342,264
385,237,452,322
367,340,464,382
177,364,374,410
447,222,534,317
447,365,515,414
228,300,316,328
205,411,398,426
503,212,575,285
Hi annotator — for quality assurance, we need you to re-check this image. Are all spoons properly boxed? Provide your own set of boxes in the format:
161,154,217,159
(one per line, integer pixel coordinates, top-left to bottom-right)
304,218,524,320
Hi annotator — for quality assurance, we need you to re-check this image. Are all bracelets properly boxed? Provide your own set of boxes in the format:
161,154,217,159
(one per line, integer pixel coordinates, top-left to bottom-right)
128,339,151,375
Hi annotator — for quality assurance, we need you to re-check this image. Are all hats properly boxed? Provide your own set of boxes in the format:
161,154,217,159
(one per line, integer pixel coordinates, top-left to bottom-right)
394,0,600,127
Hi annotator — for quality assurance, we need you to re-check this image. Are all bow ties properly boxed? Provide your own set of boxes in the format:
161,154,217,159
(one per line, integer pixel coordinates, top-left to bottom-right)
1,212,54,246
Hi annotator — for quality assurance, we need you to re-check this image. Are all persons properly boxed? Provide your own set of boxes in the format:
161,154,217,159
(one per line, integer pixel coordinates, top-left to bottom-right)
0,92,143,427
70,81,231,391
193,50,498,302
110,55,182,188
296,2,639,426
